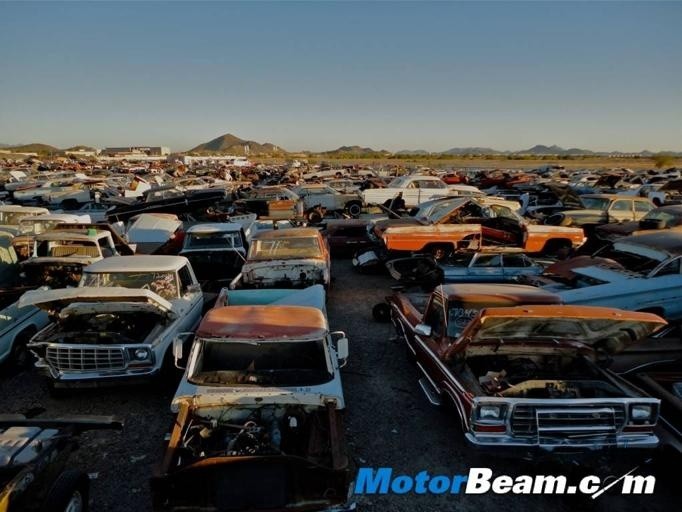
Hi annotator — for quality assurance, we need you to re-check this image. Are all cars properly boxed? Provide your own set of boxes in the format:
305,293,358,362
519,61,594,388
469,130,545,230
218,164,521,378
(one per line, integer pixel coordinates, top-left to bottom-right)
16,254,205,390
362,174,486,216
385,281,669,454
